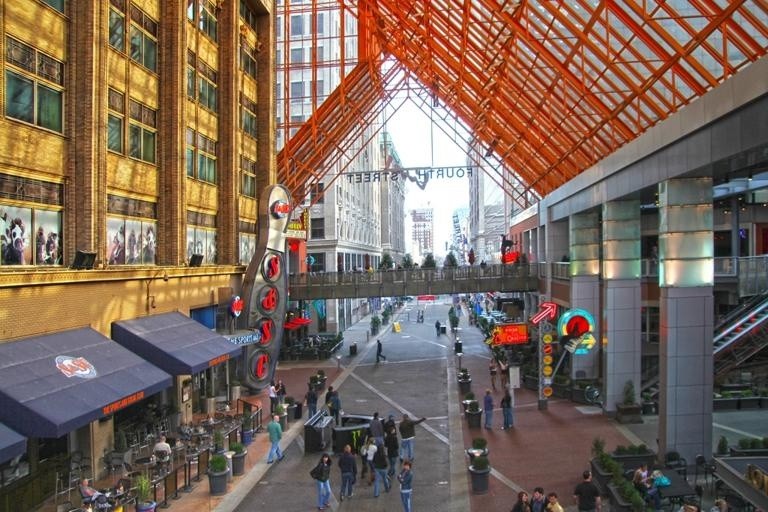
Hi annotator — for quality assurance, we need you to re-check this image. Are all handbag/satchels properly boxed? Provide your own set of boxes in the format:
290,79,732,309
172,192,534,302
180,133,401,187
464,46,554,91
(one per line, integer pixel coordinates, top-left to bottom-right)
326,399,337,409
650,470,672,487
309,464,324,480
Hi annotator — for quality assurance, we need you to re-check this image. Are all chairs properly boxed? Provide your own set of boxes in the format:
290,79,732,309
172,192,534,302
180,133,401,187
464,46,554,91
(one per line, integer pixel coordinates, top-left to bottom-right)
44,407,244,510
625,453,755,512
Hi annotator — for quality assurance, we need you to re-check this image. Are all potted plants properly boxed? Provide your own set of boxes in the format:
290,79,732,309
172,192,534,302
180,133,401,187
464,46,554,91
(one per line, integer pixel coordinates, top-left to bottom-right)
207,400,253,496
713,435,767,458
456,367,491,492
371,309,389,336
587,437,656,511
273,370,327,432
320,332,346,359
380,252,457,281
477,317,595,404
615,381,768,424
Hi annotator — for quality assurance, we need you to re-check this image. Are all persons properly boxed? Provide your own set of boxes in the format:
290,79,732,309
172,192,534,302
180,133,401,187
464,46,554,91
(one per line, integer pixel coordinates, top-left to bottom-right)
266,379,429,511
208,415,214,426
376,340,387,362
435,320,441,336
561,255,569,265
480,260,486,269
511,461,728,512
309,335,314,349
366,264,375,282
398,264,403,271
216,409,219,414
180,421,194,440
338,261,343,274
650,246,659,275
316,335,320,344
484,355,514,430
225,400,232,411
116,482,124,496
152,421,172,470
77,478,102,499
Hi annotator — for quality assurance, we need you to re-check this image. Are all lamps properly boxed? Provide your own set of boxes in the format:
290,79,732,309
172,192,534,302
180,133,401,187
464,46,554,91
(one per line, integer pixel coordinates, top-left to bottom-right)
147,269,169,287
148,295,157,309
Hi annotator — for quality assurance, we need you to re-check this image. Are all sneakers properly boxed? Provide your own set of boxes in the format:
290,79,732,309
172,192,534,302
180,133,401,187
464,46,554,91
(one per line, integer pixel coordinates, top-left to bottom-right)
386,472,393,481
279,454,284,461
324,502,331,508
266,460,274,464
348,492,354,498
318,506,326,510
340,496,344,501
